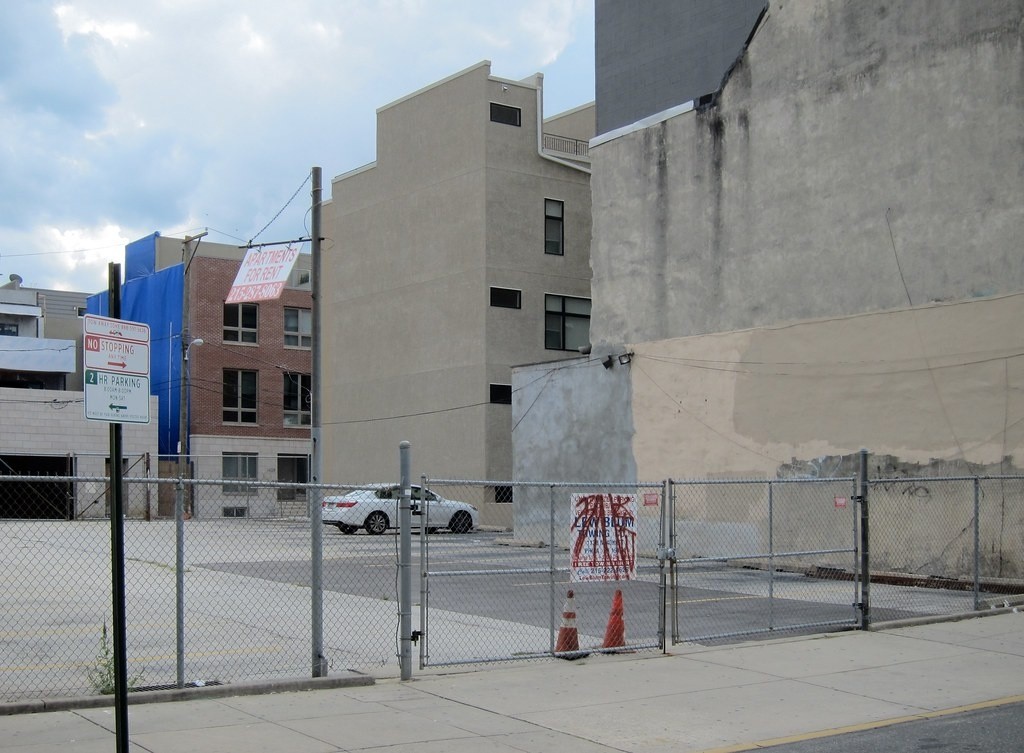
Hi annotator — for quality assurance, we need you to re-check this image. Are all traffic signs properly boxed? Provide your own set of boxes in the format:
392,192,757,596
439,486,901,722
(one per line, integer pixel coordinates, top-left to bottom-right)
83,369,150,424
83,334,150,377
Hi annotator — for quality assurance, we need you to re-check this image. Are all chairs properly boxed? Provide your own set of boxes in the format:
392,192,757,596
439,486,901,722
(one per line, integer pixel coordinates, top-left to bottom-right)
379,490,393,499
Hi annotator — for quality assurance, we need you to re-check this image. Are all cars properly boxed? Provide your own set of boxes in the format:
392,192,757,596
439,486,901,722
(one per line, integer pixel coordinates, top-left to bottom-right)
322,483,478,535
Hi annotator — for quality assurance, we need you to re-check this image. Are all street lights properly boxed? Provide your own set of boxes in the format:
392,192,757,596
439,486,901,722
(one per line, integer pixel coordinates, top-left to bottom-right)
184,338,204,464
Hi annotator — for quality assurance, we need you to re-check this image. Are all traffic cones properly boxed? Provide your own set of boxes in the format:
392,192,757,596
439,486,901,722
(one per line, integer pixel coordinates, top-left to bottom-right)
553,587,581,650
594,590,636,655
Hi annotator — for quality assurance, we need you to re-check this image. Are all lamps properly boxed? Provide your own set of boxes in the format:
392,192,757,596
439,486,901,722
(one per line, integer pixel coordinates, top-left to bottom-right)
619,352,634,365
600,355,614,369
